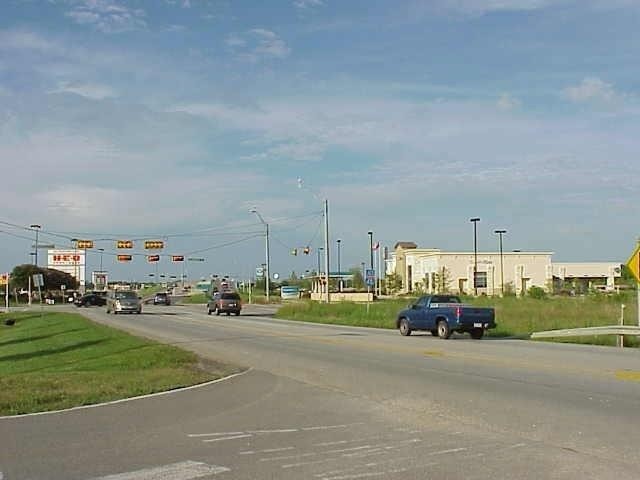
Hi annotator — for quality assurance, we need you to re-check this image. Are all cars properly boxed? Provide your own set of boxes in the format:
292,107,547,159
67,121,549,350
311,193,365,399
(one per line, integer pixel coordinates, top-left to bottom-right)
73,294,107,308
107,290,142,314
207,293,241,315
153,292,169,305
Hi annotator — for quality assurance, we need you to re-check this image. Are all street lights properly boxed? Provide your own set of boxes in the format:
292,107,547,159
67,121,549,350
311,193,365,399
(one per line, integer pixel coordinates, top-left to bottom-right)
248,210,269,302
470,218,481,295
368,231,373,271
317,247,323,275
336,240,341,275
494,230,507,294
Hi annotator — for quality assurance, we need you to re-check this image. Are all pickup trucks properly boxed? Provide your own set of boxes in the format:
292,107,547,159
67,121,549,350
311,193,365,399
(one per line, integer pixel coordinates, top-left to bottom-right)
396,294,497,339
